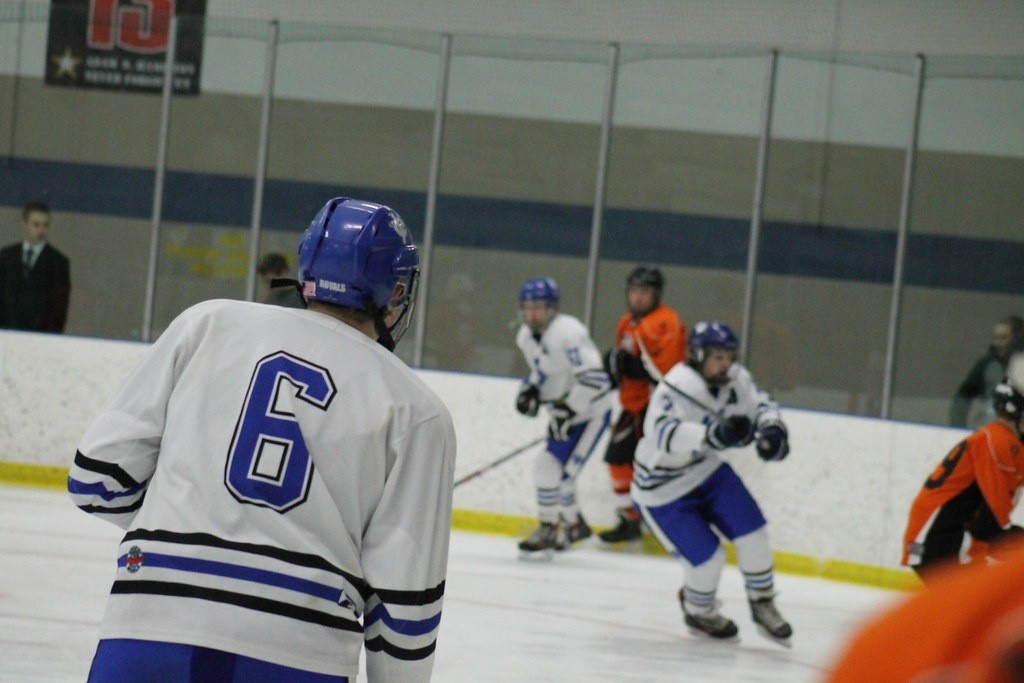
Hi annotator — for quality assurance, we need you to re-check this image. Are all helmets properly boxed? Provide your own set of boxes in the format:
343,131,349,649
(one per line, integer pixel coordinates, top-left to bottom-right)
990,375,1024,424
690,321,739,385
622,266,664,313
519,277,562,330
296,197,420,354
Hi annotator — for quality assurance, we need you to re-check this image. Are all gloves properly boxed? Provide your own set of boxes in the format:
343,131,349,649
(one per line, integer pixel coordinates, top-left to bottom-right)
712,414,755,449
755,427,788,461
606,348,632,371
518,387,539,415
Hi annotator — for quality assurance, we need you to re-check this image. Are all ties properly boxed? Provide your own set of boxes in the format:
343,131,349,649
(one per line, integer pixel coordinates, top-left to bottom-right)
24,250,34,272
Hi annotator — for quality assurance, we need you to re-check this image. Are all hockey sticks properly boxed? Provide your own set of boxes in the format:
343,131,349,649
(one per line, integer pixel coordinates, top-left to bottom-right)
637,336,770,451
454,433,547,489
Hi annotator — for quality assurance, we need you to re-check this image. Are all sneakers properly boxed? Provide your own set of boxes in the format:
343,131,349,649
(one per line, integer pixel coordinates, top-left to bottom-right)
518,524,556,561
748,598,793,650
598,519,642,550
686,610,739,644
556,517,591,550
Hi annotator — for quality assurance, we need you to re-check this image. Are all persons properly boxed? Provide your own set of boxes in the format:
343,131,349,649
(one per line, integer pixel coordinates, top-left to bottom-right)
629,321,796,640
0,197,73,338
597,264,685,543
62,191,457,683
261,253,308,309
896,370,1023,587
509,277,613,560
947,317,1023,431
